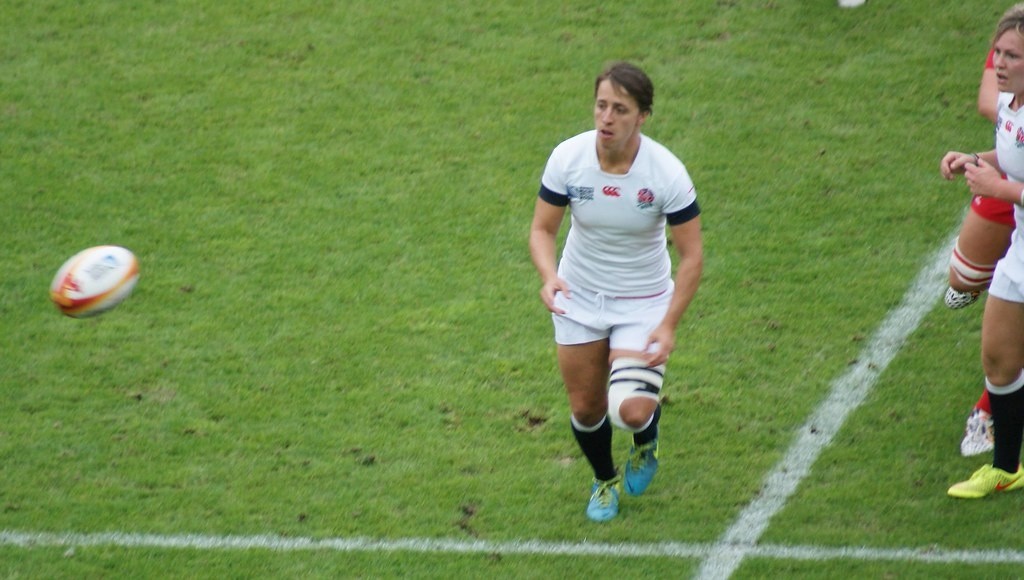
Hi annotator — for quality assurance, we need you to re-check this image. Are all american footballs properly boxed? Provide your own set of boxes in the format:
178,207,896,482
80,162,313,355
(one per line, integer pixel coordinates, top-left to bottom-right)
48,243,140,317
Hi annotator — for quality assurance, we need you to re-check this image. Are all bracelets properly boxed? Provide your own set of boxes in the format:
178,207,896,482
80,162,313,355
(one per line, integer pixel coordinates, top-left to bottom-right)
972,152,980,166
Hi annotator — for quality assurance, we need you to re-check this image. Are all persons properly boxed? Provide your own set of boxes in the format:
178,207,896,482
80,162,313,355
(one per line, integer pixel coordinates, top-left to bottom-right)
529,62,704,520
941,3,1024,500
944,39,1017,457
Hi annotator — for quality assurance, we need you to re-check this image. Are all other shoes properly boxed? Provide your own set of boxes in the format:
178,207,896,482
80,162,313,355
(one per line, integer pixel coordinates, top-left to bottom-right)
961,388,995,457
945,286,984,310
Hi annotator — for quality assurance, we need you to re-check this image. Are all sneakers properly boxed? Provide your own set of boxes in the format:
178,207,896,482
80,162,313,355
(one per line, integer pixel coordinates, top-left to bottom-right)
587,476,622,522
947,463,1024,498
625,433,659,495
